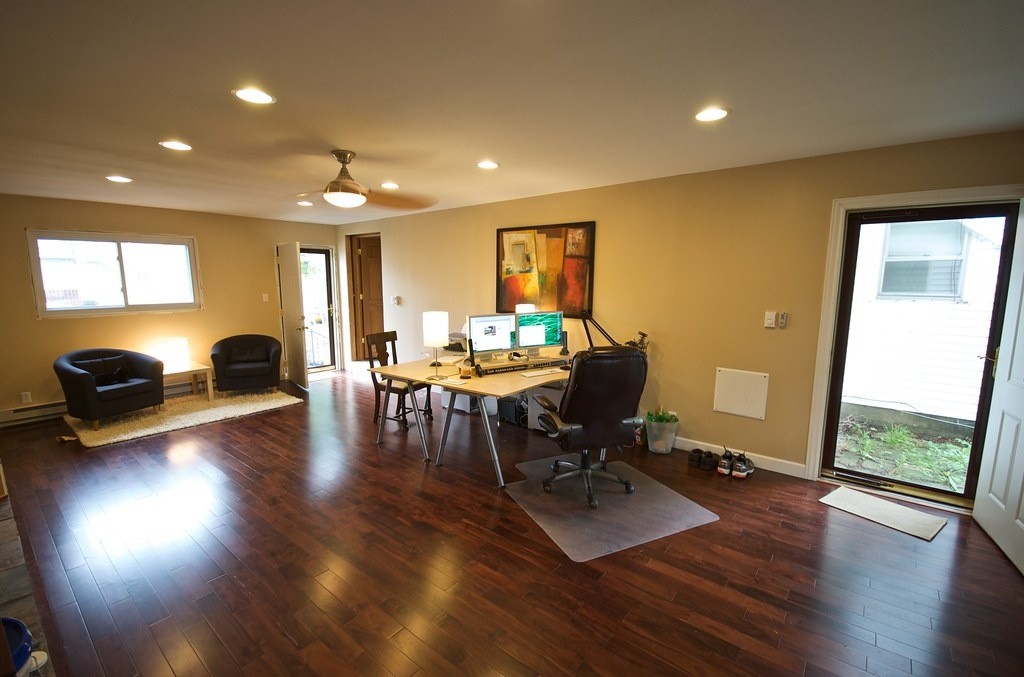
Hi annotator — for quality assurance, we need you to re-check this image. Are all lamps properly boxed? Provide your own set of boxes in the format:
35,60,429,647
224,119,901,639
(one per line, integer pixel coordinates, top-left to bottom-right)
423,311,450,381
515,304,536,359
579,307,650,359
321,150,367,209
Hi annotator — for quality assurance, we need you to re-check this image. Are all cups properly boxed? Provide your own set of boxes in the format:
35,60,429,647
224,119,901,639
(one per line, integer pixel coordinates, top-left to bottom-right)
461,361,472,379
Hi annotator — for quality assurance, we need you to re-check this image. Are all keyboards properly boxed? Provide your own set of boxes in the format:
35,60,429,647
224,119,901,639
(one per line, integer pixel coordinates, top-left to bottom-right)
521,368,564,377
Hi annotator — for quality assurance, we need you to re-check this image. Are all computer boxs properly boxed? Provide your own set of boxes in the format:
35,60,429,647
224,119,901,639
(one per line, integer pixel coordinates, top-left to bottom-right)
441,386,479,414
528,386,567,431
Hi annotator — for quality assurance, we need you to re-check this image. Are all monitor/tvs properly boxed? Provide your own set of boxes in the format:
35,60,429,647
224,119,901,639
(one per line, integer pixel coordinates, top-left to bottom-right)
469,313,516,364
516,310,563,359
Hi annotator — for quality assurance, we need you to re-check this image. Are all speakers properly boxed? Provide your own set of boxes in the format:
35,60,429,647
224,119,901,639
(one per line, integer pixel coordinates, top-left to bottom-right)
559,331,569,355
468,339,475,367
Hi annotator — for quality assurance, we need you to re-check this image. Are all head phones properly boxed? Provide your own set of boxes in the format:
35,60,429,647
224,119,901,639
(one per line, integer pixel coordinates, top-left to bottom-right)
508,352,529,362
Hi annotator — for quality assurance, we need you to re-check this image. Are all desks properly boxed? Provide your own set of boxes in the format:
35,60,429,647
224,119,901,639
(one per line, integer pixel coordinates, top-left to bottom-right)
158,360,214,412
366,346,552,461
426,357,573,487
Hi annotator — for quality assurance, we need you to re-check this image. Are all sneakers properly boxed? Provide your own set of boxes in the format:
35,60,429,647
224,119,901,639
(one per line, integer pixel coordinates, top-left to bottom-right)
731,449,754,478
716,446,736,475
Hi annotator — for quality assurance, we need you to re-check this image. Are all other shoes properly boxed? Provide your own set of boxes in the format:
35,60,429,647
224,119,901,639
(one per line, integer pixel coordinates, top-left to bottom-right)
688,449,704,467
700,451,721,471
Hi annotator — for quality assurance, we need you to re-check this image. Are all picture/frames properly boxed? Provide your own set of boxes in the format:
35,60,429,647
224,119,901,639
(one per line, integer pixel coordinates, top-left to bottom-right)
496,221,596,320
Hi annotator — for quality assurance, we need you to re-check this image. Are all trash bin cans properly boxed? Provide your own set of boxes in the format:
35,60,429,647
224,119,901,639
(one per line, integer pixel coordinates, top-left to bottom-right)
646,417,678,454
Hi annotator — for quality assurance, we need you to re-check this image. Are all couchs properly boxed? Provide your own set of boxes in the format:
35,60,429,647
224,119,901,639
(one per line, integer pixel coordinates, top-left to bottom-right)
52,348,164,432
209,334,282,398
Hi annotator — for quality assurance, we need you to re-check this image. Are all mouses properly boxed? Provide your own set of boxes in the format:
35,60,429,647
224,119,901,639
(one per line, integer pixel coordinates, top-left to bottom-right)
560,366,571,370
430,361,442,366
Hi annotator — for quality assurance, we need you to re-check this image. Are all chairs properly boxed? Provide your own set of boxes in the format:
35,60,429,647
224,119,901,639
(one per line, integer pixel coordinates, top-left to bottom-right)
533,346,649,510
361,331,434,432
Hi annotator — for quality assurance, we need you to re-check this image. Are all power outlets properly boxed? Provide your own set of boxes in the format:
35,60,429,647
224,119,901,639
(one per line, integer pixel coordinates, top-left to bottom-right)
20,392,32,404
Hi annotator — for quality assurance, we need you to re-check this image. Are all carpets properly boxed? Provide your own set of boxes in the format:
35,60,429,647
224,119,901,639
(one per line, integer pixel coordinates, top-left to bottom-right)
503,453,721,563
818,485,950,543
63,387,305,447
